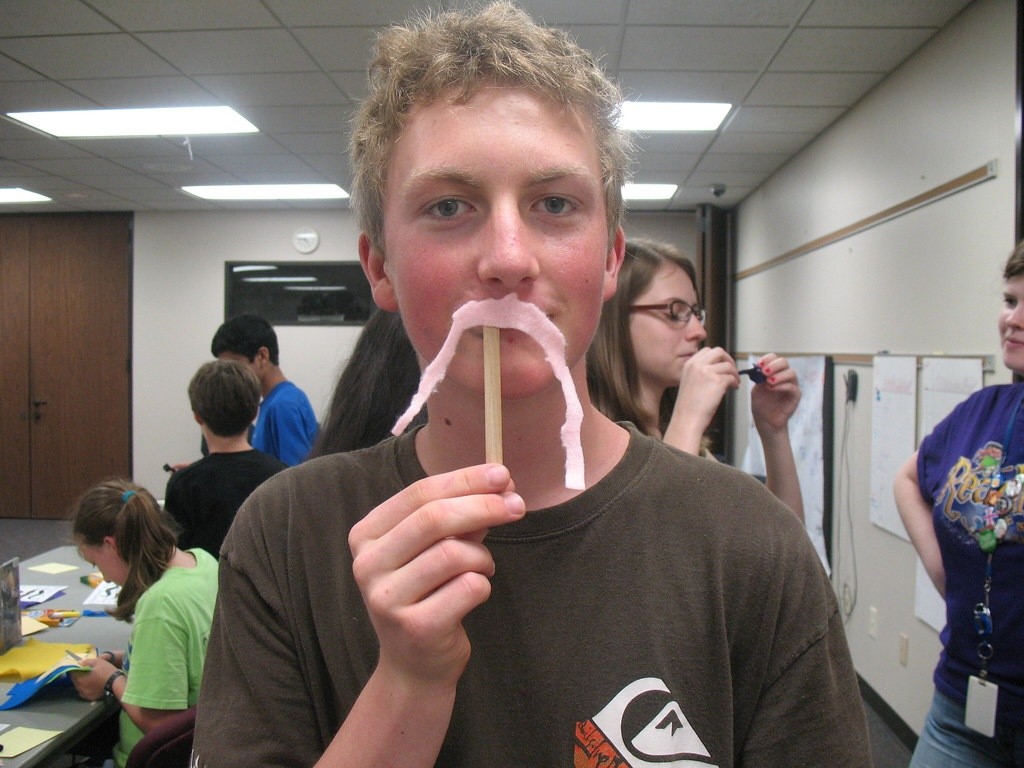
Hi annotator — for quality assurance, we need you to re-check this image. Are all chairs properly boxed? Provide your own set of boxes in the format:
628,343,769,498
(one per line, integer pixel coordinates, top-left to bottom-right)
125,704,197,768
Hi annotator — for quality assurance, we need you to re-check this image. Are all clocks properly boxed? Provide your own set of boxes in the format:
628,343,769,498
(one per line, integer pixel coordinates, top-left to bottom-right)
292,225,319,252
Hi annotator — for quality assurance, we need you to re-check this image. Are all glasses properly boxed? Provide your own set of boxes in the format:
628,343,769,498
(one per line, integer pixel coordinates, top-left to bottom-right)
627,300,708,328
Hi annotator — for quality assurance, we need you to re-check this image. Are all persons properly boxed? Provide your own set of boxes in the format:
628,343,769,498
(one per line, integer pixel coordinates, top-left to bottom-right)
163,358,292,560
210,316,321,469
191,0,874,768
307,307,431,463
892,238,1024,767
67,481,218,768
585,239,804,532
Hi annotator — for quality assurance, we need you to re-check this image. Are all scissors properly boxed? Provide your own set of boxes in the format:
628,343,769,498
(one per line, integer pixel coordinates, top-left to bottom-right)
67,650,115,665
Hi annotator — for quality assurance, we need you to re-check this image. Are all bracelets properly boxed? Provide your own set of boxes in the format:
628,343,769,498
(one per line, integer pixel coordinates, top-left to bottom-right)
104,651,115,662
105,670,124,696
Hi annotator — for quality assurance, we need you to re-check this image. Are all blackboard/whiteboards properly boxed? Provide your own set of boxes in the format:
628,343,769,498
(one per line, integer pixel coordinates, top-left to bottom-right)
269,319,366,430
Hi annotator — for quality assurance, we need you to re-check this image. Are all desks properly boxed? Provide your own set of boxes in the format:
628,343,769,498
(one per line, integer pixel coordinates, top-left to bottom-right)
0,545,134,768
